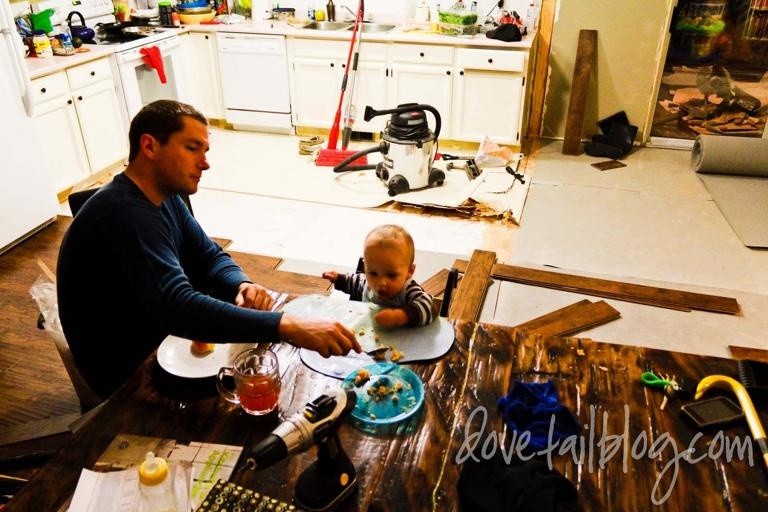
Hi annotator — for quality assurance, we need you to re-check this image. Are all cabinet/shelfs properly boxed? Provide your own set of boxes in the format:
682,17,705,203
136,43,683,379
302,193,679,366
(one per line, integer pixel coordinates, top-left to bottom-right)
454,26,537,154
218,15,303,137
31,0,116,39
18,41,130,204
388,21,457,148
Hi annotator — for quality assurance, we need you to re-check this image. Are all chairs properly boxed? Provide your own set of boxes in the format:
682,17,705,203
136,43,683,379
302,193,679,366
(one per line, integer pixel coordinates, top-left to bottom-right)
346,256,456,318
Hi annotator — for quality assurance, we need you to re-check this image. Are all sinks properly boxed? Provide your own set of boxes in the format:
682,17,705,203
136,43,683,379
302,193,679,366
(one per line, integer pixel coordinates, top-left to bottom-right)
348,24,395,34
301,22,349,32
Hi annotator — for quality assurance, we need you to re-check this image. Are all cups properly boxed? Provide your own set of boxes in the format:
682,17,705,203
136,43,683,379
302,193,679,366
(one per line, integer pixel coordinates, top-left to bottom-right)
315,10,325,22
215,348,281,417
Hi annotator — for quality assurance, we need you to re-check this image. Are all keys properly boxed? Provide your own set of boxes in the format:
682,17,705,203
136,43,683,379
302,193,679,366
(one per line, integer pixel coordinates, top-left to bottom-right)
641,371,682,410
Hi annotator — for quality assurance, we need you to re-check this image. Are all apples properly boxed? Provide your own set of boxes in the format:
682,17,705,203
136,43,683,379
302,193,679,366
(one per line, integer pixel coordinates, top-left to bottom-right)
315,10,325,20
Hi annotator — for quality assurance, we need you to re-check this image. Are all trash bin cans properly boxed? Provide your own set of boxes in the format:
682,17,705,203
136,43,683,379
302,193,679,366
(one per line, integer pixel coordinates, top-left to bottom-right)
677,20,726,58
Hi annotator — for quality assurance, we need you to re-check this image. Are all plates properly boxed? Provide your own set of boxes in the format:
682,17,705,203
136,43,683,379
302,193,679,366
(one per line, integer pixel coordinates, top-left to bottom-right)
157,335,263,378
343,363,425,425
299,347,395,380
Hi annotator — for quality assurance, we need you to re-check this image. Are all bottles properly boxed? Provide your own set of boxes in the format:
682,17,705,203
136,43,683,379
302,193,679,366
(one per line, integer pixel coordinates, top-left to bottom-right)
158,3,180,27
139,451,174,512
33,30,53,59
326,0,336,22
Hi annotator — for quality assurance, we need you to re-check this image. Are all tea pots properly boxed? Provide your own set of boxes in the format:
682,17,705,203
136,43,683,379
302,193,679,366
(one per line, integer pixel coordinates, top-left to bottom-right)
66,11,95,43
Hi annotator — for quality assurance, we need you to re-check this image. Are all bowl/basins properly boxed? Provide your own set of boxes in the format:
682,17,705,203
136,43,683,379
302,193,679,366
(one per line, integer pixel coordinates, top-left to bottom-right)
177,1,217,25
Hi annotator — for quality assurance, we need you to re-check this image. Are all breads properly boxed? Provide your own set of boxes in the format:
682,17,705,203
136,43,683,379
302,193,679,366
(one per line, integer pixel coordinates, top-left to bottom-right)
192,341,215,353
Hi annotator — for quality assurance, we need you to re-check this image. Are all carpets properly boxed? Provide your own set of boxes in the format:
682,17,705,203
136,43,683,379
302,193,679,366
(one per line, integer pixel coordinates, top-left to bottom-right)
198,126,536,225
690,133,767,248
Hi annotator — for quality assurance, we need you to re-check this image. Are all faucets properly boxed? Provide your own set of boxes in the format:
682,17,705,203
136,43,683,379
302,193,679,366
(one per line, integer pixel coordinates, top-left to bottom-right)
340,5,357,18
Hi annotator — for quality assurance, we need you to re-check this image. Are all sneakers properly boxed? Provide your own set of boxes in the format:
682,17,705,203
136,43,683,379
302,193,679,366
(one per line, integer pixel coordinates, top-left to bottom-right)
298,135,326,156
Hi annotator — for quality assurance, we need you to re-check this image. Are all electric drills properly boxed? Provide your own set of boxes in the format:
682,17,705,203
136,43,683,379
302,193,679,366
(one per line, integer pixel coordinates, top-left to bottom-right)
237,387,358,511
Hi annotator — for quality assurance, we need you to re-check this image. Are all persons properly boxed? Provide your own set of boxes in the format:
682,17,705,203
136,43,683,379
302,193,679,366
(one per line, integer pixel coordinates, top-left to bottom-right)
322,225,436,330
56,100,361,400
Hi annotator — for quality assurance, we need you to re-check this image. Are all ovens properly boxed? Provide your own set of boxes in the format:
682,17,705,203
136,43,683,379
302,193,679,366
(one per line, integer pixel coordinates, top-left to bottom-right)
115,35,187,122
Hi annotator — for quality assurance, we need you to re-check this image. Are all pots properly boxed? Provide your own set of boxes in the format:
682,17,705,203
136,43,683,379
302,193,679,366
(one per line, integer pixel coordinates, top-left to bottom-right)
96,22,137,34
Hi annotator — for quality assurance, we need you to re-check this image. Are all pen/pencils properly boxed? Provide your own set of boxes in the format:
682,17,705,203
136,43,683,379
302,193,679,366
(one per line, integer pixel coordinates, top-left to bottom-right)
720,460,734,512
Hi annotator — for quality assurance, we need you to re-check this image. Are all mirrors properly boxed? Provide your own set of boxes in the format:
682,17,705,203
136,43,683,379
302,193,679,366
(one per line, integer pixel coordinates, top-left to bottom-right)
641,0,766,151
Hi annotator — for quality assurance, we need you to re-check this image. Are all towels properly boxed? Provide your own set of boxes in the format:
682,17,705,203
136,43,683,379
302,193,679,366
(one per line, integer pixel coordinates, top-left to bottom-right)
140,46,166,83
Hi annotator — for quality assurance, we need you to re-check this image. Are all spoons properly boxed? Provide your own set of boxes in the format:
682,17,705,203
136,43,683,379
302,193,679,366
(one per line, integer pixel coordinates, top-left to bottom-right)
351,347,389,357
